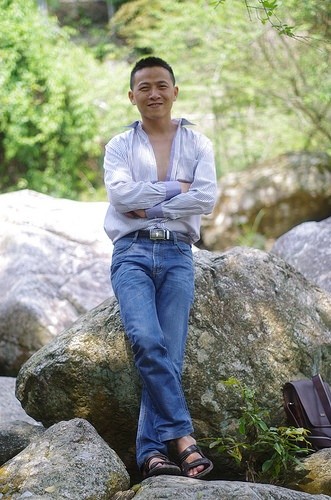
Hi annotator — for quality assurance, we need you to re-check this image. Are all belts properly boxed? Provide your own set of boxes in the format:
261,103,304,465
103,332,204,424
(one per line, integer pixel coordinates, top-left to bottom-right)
124,229,191,245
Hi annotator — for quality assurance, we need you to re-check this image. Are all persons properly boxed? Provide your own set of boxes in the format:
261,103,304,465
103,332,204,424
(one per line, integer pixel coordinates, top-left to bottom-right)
104,57,218,478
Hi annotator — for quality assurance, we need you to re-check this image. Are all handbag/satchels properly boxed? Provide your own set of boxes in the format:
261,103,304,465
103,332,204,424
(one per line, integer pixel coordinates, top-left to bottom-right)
283,374,331,448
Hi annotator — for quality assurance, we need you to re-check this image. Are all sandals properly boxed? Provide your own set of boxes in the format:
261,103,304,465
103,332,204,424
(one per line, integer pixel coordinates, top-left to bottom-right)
176,444,213,479
141,453,181,479
